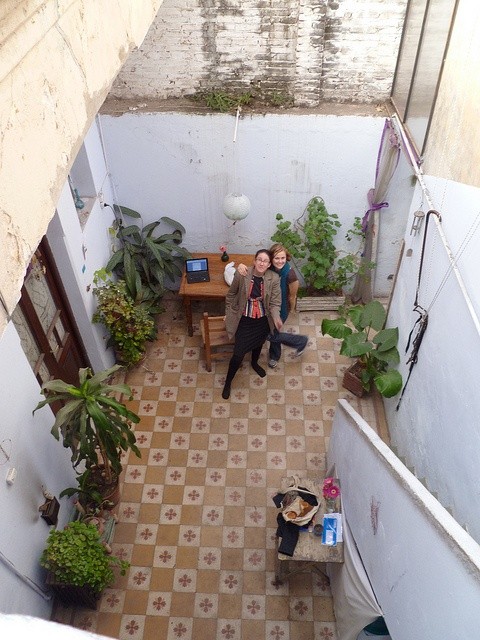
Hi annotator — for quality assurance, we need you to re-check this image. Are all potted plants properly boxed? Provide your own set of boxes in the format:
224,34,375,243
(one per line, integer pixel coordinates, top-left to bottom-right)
39,520,130,611
321,300,402,398
32,367,141,510
72,501,121,554
86,267,154,368
269,196,377,312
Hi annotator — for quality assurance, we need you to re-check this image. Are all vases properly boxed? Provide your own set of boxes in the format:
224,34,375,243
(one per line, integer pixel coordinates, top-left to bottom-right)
222,251,229,261
73,188,85,209
313,524,324,534
41,489,54,500
327,508,336,513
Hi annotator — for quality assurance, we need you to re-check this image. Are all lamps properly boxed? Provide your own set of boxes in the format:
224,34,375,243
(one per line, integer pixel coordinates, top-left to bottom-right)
223,192,251,225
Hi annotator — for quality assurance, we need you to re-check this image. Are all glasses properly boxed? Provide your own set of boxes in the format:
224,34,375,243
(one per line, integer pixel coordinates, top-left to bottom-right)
255,259,270,265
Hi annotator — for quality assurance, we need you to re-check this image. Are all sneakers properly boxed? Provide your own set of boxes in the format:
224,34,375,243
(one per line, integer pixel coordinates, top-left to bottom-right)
268,358,278,367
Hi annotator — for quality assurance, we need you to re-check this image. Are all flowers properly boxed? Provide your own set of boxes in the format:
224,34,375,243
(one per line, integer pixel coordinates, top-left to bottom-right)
219,246,227,252
322,479,340,507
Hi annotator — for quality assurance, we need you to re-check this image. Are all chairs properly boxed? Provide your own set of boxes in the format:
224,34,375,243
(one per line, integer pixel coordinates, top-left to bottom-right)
199,311,237,372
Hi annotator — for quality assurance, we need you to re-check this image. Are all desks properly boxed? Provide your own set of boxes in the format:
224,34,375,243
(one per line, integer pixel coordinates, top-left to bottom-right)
272,478,344,585
178,252,259,338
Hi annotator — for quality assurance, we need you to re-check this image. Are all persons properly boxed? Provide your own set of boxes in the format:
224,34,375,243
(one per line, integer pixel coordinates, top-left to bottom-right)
237,243,310,369
221,249,284,399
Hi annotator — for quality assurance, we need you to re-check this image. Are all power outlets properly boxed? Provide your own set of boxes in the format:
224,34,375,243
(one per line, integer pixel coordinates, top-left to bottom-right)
100,202,108,208
5,467,18,484
